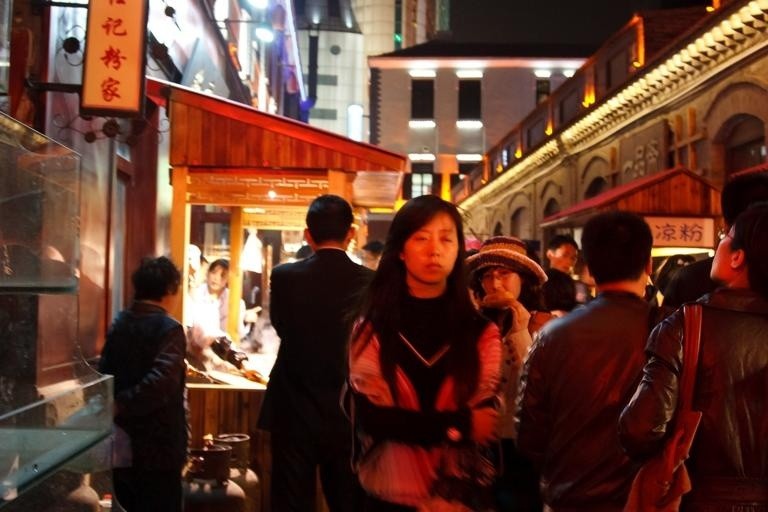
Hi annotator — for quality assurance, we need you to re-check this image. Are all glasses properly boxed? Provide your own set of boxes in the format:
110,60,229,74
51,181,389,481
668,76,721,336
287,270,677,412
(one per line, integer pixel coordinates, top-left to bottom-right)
717,228,734,242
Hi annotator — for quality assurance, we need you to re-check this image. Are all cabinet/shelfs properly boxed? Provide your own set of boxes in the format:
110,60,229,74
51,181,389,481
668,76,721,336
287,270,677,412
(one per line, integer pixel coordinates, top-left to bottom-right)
186,380,327,512
1,112,118,511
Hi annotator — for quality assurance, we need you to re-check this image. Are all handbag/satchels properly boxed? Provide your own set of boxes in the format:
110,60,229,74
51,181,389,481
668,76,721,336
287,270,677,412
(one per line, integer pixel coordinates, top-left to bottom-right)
619,300,705,512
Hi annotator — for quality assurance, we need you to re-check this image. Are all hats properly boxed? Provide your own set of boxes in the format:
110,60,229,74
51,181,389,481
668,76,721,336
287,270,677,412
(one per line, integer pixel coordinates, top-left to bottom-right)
463,235,549,291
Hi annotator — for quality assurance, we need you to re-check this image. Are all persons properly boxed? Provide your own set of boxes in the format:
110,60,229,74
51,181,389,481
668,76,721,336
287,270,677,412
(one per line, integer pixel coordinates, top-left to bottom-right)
95,254,186,510
191,258,244,348
258,168,768,511
